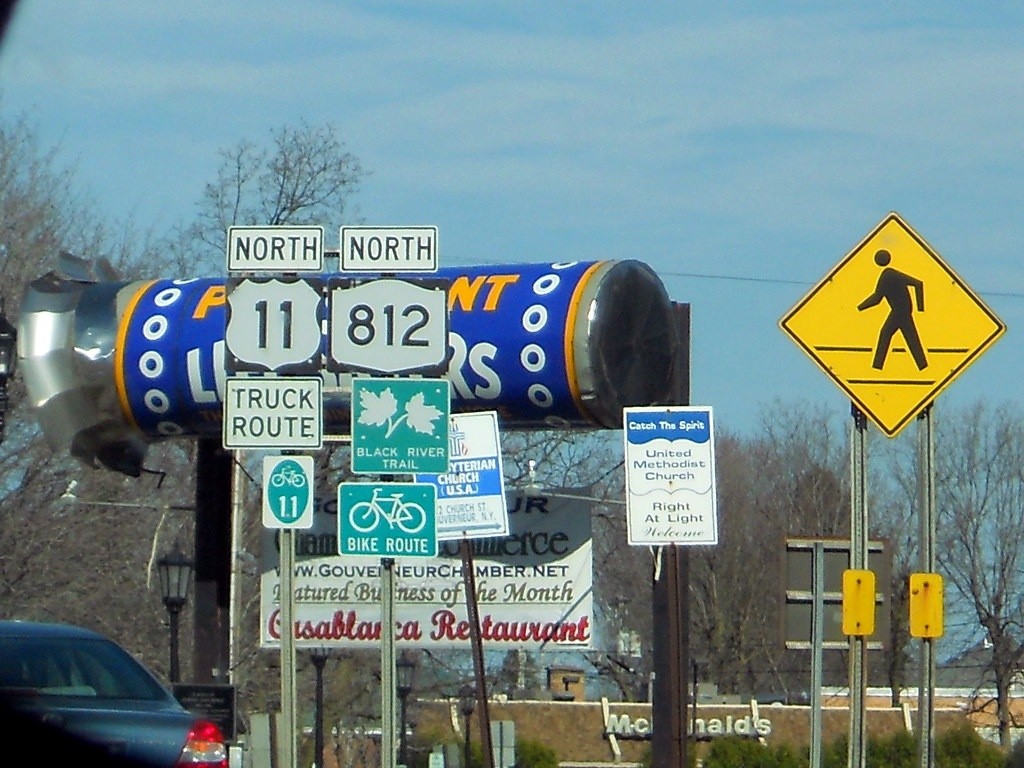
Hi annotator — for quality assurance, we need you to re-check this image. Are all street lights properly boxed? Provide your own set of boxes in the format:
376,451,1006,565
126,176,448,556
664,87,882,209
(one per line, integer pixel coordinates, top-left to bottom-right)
396,648,415,768
155,537,196,684
304,646,333,768
457,682,477,768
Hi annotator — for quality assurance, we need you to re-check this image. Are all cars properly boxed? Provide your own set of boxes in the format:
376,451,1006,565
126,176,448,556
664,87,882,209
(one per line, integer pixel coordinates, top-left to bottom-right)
0,619,227,768
